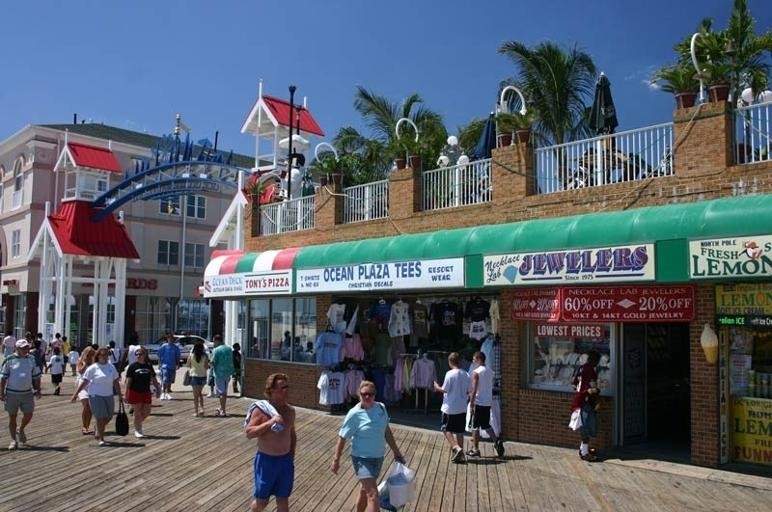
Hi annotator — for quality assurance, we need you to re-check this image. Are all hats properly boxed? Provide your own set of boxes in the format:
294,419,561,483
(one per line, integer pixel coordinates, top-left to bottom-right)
15,339,30,348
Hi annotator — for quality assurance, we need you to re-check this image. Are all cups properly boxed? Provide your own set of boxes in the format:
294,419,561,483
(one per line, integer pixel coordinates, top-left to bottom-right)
747,371,755,388
702,344,719,364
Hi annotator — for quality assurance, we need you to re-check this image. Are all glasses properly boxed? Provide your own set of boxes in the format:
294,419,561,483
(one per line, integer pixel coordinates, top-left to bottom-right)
135,353,143,356
360,392,375,396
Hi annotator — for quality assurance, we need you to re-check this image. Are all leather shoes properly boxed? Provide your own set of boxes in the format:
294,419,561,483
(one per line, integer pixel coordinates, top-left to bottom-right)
88,429,95,434
82,429,90,434
98,441,104,446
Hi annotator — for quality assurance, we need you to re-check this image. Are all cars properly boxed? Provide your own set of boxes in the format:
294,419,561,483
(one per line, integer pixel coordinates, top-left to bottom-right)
142,334,214,364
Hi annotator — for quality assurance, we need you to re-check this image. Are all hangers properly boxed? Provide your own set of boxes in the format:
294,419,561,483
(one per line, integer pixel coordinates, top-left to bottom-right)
469,296,488,304
328,366,340,372
347,361,362,371
323,325,336,334
345,331,354,338
436,301,458,307
412,300,427,307
378,299,386,304
330,299,361,304
416,353,429,360
400,353,417,357
394,297,406,303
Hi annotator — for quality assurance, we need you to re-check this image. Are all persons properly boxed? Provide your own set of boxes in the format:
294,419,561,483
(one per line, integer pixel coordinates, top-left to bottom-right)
60,336,71,372
245,373,297,512
30,340,47,395
465,351,505,457
233,343,241,393
68,346,79,376
37,333,47,352
206,360,215,398
209,334,235,417
74,346,96,435
331,381,406,512
124,349,161,437
158,334,180,400
183,341,210,416
0,339,42,450
249,331,315,364
45,347,64,395
52,333,62,349
24,331,33,343
571,350,605,462
108,341,122,396
434,351,475,464
71,348,123,446
2,330,16,358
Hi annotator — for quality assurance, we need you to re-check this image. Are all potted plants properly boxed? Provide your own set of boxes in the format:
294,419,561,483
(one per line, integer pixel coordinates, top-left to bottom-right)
512,111,536,144
402,140,425,168
312,162,328,187
649,64,699,111
495,112,517,148
389,141,406,170
328,156,343,185
699,52,735,102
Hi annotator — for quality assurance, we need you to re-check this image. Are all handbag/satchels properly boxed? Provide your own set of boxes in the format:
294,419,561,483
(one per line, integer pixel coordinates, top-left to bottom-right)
183,370,191,385
115,399,129,436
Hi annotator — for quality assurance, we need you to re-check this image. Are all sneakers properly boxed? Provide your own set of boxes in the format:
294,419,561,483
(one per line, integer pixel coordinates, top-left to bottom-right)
160,394,166,400
16,426,26,443
217,412,226,417
165,394,172,400
135,430,143,438
494,438,505,458
8,440,18,451
465,450,481,458
459,454,466,463
450,445,463,462
